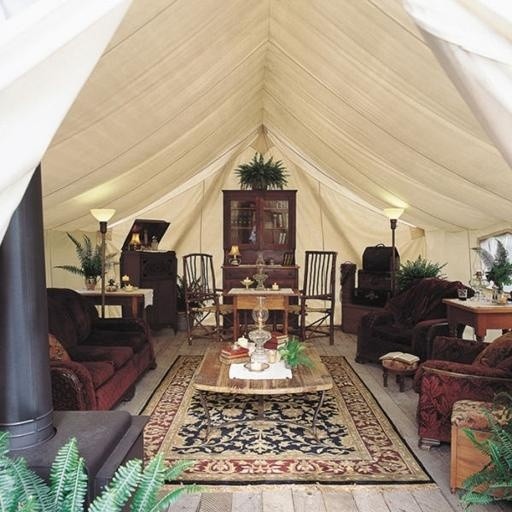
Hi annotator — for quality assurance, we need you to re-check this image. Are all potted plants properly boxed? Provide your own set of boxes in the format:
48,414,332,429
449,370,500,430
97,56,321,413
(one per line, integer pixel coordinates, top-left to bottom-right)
53,231,118,291
470,239,512,303
234,152,291,190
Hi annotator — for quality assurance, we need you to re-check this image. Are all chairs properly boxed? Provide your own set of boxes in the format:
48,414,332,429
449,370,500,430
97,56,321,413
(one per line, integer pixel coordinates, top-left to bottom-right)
416,331,512,451
289,250,337,345
182,253,233,345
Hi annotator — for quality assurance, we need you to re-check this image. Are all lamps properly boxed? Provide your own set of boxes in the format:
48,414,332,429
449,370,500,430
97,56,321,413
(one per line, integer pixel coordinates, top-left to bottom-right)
228,245,242,266
90,208,117,319
384,208,404,313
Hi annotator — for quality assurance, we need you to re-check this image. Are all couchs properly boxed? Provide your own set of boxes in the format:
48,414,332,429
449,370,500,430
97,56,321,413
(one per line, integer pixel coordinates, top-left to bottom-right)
47,288,157,411
356,278,475,366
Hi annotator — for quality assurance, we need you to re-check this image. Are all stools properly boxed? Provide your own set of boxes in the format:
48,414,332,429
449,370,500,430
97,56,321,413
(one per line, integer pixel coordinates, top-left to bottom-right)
382,360,418,392
450,400,512,499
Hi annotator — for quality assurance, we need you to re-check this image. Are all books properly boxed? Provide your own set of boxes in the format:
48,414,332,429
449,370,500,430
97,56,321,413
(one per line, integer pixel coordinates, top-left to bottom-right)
273,214,283,228
231,210,256,227
279,232,286,245
283,252,294,265
379,352,420,365
218,345,251,365
264,332,289,350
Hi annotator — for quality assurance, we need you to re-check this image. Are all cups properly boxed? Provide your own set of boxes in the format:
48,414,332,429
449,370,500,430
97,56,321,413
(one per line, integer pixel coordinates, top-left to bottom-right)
497,294,507,305
458,289,467,301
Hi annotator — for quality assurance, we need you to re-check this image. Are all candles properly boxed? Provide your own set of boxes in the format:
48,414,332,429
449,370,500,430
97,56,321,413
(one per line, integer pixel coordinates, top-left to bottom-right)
243,277,251,282
122,274,129,281
272,282,279,289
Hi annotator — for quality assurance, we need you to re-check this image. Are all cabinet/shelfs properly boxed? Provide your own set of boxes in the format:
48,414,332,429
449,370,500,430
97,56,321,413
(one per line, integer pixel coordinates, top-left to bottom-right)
221,189,299,329
120,250,178,335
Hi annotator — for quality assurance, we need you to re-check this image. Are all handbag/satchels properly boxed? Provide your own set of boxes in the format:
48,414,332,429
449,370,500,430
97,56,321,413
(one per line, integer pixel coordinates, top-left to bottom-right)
362,244,400,271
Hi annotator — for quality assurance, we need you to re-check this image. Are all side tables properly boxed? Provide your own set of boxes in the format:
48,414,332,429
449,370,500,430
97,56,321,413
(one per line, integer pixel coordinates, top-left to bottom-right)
225,287,299,341
442,297,512,343
75,288,153,320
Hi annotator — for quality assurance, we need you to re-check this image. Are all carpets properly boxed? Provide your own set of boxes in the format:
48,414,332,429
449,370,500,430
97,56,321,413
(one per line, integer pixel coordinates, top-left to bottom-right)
137,356,435,487
